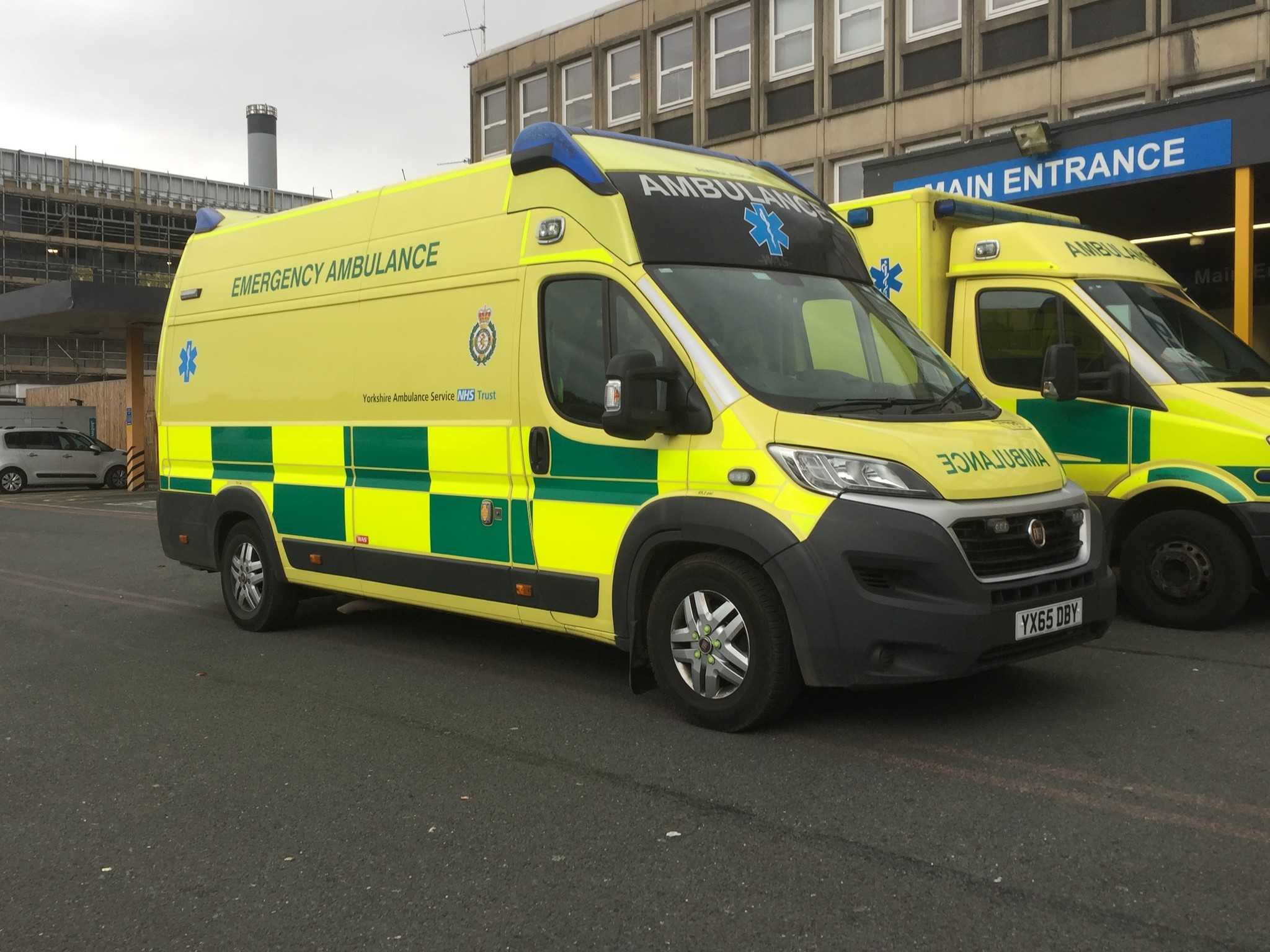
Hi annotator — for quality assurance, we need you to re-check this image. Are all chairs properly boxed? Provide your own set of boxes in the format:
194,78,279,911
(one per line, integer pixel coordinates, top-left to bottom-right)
570,297,630,402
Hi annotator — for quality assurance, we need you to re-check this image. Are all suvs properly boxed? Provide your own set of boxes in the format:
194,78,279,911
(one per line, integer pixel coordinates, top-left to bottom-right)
0,425,128,494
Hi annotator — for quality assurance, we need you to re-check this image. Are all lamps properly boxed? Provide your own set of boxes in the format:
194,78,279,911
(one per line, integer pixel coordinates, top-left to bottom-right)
1011,120,1065,158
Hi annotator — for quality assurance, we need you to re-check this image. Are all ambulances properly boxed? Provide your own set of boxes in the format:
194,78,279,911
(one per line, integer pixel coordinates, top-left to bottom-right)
758,183,1270,632
152,119,1120,734
